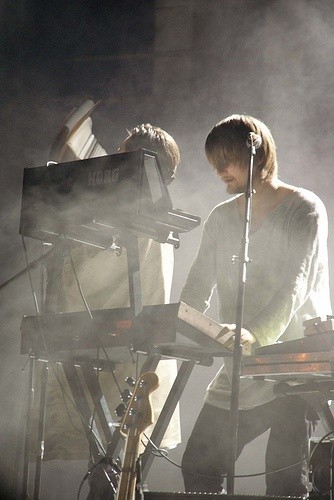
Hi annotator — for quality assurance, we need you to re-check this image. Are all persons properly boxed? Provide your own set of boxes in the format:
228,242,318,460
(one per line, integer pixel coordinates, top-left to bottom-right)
175,114,332,499
62,124,180,460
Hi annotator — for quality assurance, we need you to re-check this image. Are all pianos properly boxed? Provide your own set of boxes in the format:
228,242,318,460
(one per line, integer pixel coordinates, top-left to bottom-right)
20,307,251,360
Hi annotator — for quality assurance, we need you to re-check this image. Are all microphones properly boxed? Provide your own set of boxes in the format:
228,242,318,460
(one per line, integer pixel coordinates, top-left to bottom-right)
246,132,262,150
